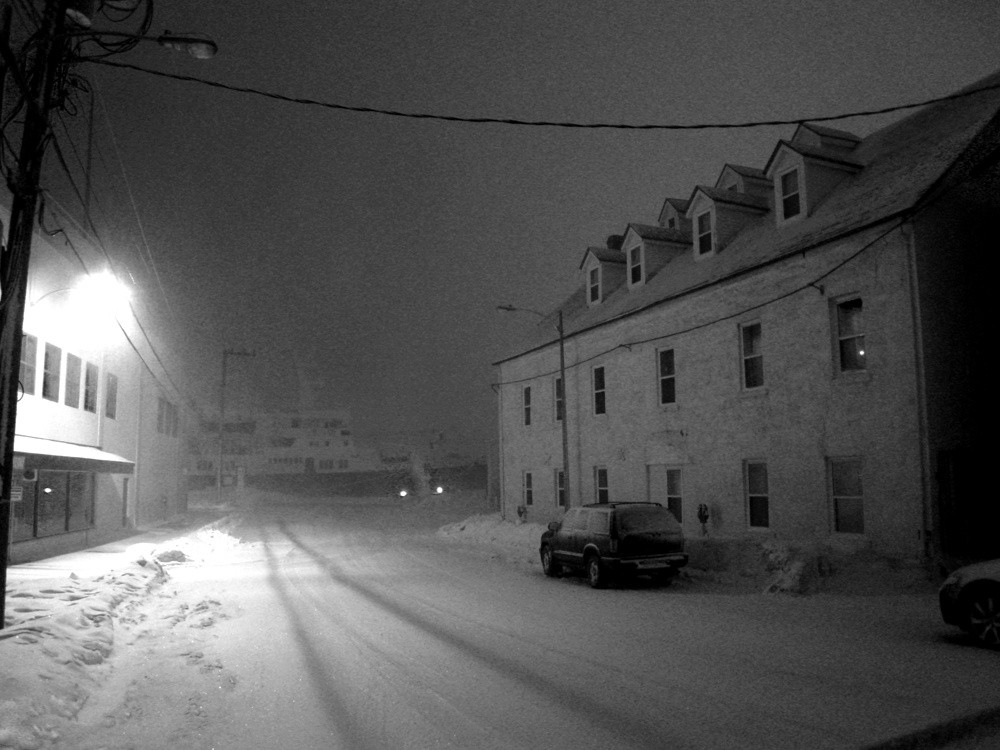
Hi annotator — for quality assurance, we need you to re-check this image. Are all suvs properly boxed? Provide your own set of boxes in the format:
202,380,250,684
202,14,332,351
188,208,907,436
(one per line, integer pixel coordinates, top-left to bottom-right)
539,500,690,590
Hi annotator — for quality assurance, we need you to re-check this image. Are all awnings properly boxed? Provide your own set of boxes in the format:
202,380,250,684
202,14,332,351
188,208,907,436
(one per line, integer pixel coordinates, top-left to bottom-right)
14,436,135,475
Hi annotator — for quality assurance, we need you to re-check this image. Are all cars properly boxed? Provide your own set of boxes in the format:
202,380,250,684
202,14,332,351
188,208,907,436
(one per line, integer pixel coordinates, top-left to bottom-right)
937,558,1000,647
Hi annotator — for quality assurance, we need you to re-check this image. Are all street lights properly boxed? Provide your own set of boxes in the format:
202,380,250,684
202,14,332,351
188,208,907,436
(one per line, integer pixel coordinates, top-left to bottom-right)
2,23,221,630
214,346,259,498
494,304,572,511
